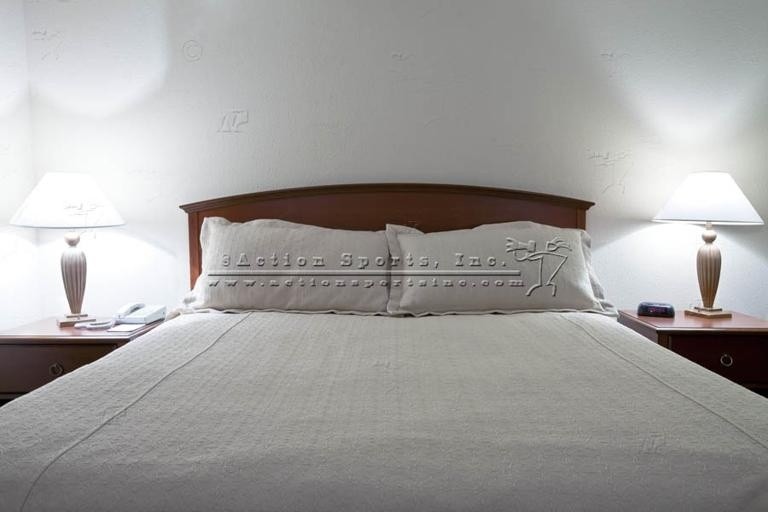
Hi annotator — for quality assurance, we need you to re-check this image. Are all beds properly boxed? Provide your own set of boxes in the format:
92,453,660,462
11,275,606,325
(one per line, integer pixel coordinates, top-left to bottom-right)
0,183,768,512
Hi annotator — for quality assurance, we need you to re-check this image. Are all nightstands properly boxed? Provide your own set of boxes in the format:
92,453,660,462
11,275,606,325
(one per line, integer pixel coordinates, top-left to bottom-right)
617,307,768,394
0,317,161,404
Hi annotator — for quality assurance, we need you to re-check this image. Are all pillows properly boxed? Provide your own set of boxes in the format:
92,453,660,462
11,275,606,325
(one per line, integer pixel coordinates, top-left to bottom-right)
168,215,404,323
385,221,619,318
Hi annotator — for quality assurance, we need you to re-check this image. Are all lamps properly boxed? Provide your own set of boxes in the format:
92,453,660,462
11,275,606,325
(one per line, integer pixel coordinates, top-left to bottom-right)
9,172,126,329
651,172,764,319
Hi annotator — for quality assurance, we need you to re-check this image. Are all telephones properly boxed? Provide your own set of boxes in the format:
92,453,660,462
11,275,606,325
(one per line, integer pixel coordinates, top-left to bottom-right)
113,302,167,324
